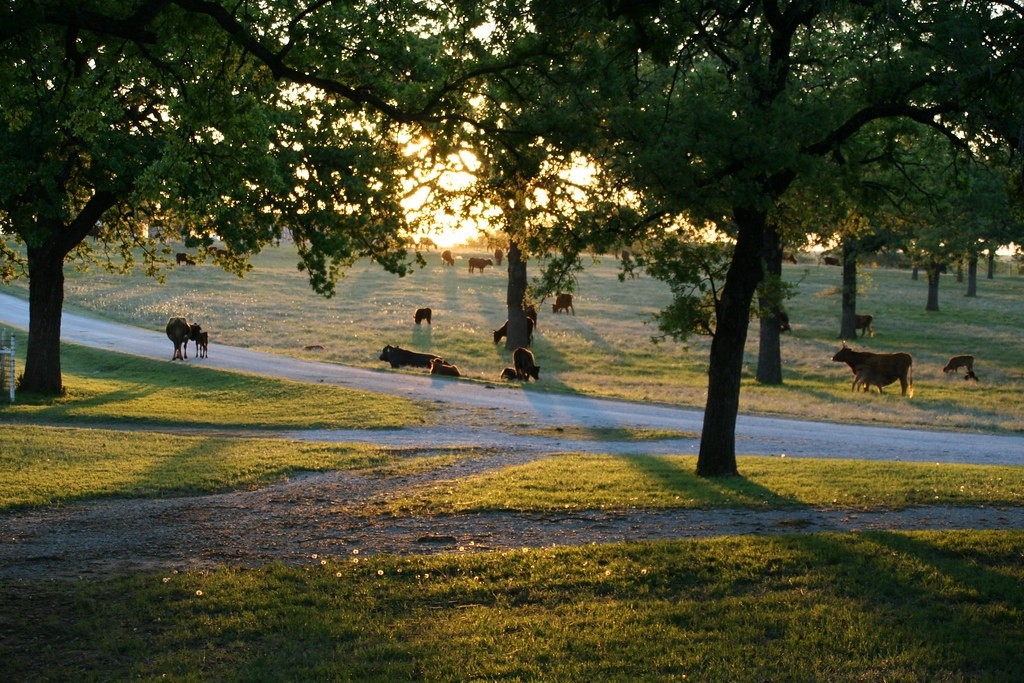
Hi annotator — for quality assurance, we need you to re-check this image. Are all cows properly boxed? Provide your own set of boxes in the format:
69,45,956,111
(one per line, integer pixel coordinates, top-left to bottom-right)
441,250,454,266
782,252,797,265
176,252,195,265
551,294,575,316
831,340,914,399
379,344,451,368
943,355,974,375
494,318,533,347
854,314,873,338
469,257,493,273
208,247,217,253
494,250,503,266
823,256,842,266
166,317,208,361
216,249,231,260
413,308,432,324
501,368,528,381
430,357,461,377
512,347,540,380
